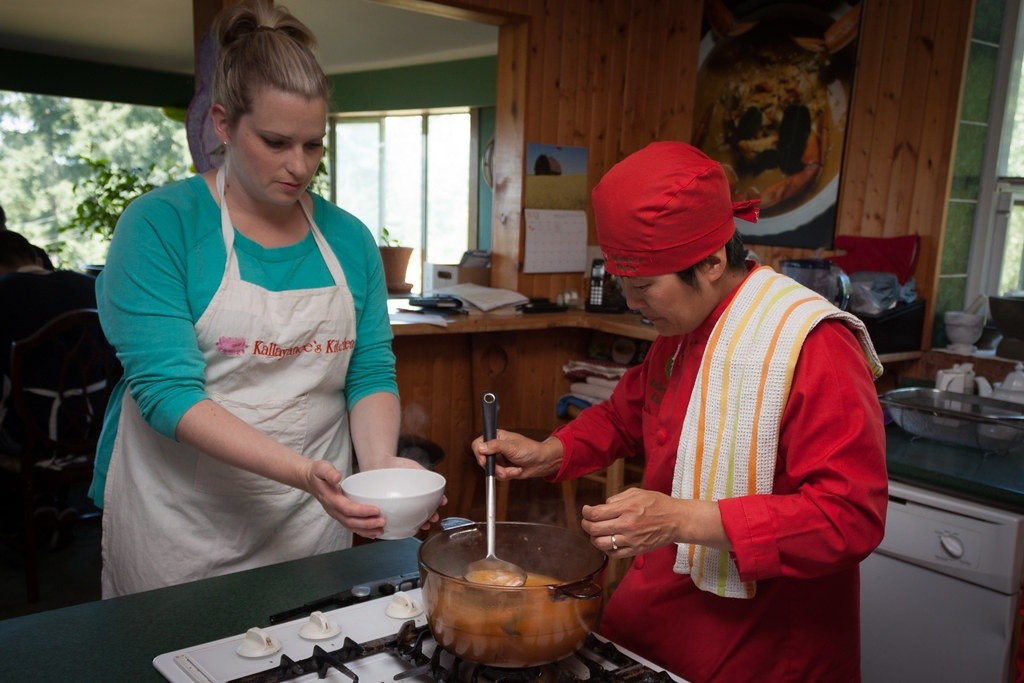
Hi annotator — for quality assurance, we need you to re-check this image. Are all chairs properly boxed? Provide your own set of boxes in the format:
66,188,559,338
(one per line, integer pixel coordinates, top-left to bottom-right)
0,308,126,606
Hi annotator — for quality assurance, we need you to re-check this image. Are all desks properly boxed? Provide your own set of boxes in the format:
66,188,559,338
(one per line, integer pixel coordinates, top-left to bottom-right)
391,298,921,366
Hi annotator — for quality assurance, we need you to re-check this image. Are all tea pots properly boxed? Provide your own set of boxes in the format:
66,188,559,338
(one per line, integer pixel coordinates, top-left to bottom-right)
974,363,1024,405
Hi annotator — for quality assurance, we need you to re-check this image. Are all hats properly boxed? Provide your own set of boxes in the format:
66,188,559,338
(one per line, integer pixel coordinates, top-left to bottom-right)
591,141,761,277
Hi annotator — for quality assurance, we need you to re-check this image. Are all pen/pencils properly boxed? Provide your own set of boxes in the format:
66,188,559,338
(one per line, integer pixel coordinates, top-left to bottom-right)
396,306,423,315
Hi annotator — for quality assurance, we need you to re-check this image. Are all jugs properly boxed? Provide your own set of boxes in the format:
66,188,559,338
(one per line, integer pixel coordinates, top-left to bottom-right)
778,258,852,310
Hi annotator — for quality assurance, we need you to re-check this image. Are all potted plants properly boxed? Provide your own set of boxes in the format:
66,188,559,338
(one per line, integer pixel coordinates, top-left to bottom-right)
46,153,174,277
379,227,414,291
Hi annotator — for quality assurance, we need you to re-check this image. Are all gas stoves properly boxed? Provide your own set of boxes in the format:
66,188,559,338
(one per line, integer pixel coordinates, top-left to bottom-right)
151,571,685,683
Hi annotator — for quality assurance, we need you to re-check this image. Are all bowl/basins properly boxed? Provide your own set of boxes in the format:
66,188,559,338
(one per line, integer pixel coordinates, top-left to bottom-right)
341,467,446,540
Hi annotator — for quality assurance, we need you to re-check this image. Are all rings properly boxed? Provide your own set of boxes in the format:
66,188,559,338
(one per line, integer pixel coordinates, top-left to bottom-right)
612,535,620,553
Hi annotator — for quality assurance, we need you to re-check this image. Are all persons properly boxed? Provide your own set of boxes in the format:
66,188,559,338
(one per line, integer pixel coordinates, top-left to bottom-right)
88,0,447,600
0,206,54,274
469,142,889,683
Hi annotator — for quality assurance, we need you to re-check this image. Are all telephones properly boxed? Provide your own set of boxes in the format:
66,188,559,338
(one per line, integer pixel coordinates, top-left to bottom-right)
585,257,628,312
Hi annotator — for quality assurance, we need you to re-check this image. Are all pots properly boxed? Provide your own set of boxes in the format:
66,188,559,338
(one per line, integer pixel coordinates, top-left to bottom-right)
418,517,608,668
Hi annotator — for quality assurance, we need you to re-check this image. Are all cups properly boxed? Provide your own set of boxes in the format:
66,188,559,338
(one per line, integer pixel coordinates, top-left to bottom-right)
935,369,975,395
944,311,986,353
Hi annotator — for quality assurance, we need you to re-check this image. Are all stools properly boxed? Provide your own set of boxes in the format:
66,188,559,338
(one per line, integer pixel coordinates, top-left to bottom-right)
460,426,553,531
555,404,644,532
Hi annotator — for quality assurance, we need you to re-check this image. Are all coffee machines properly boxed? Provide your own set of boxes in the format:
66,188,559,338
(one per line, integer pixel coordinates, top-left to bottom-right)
988,296,1024,361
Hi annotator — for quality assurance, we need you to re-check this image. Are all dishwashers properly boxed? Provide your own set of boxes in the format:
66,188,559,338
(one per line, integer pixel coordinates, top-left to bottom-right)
860,475,1023,683
